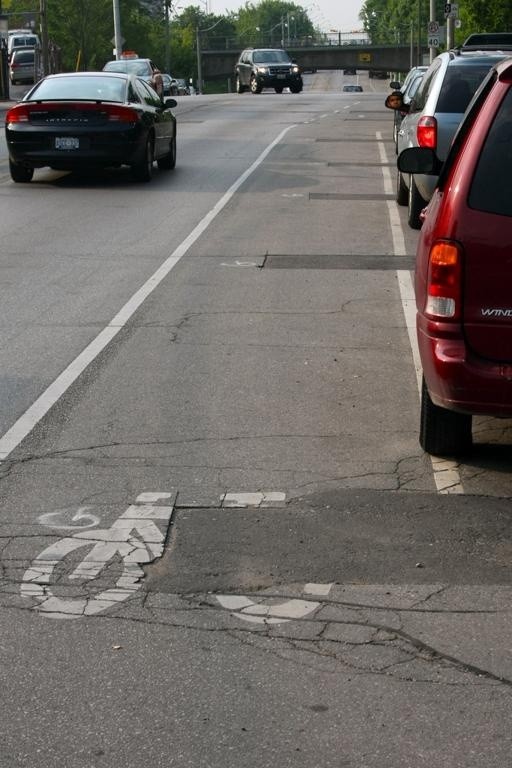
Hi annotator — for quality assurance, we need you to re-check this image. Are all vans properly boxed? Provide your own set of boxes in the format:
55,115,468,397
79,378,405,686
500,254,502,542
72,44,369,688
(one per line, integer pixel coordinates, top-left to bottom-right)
7,34,40,58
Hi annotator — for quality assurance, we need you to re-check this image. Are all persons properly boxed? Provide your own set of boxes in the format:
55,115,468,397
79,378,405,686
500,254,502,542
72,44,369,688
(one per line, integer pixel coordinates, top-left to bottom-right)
388,96,401,107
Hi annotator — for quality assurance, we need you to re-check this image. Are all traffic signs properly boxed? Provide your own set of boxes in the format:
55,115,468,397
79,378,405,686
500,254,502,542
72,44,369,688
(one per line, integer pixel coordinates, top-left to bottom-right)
427,34,440,48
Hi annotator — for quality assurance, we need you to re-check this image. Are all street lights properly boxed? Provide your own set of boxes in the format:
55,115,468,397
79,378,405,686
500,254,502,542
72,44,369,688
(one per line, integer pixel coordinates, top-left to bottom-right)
226,26,262,91
277,1,334,44
196,13,240,95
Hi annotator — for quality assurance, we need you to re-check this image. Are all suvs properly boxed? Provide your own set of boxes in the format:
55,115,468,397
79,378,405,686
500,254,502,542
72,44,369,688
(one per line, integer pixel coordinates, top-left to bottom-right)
390,50,512,461
385,25,512,229
230,45,305,94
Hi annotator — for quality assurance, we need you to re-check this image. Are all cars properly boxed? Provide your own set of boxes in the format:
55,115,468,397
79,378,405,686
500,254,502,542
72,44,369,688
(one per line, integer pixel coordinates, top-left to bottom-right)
2,68,179,184
368,70,387,79
102,50,167,103
341,84,364,92
6,28,32,37
8,49,34,85
388,63,430,155
302,69,317,73
341,69,359,75
160,73,191,96
